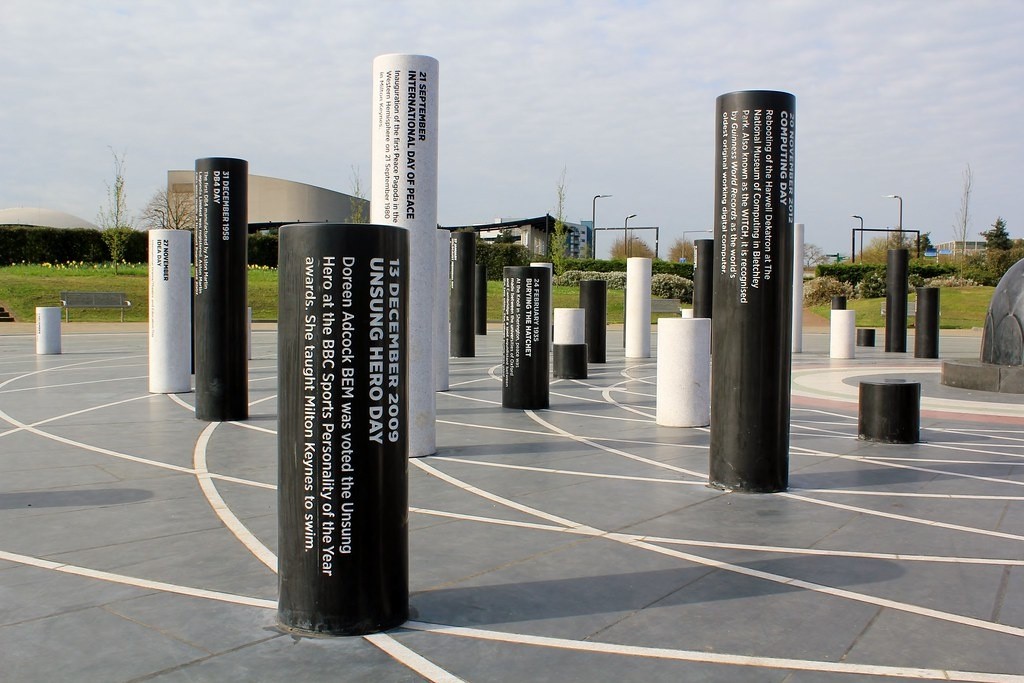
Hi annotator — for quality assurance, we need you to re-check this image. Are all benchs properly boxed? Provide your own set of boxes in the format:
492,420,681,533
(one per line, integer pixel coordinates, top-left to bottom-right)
881,302,942,325
651,299,681,323
60,292,131,323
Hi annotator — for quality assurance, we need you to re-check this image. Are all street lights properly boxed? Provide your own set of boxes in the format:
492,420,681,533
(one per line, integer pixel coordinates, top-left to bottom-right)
153,208,166,229
886,194,903,248
591,193,614,229
627,236,640,257
681,229,713,264
624,214,636,255
852,215,864,264
